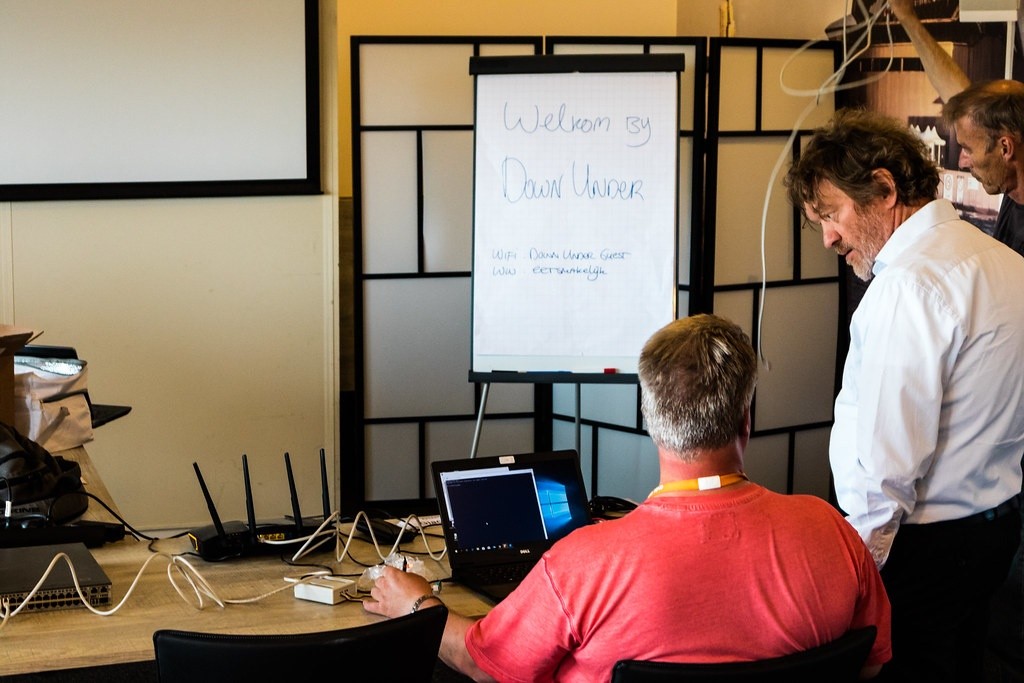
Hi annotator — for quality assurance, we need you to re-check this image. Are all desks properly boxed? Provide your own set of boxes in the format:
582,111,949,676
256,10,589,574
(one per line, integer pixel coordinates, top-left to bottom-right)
1,509,640,683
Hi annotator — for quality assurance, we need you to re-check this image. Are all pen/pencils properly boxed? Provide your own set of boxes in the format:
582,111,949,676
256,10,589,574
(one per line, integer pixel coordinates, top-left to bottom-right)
525,371,572,373
491,370,519,373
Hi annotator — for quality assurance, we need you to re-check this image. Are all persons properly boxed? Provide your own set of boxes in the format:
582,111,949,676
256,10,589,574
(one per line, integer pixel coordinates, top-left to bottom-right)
779,109,1024,683
943,80,1024,258
363,312,892,683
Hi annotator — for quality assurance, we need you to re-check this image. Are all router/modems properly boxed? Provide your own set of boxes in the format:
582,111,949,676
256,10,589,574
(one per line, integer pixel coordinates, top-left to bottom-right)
187,448,338,562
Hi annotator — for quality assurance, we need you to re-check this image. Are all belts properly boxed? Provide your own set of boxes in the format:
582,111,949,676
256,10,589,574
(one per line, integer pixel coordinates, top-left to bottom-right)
898,492,1024,542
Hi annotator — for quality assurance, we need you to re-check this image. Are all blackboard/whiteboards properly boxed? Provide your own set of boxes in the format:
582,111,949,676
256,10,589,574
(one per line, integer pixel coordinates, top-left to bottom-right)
468,52,685,384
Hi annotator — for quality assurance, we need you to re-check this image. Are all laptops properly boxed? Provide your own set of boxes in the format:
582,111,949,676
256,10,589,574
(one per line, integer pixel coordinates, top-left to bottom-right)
432,449,596,604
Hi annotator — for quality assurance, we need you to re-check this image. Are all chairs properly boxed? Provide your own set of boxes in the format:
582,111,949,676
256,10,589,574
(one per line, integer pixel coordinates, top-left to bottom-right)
153,605,449,683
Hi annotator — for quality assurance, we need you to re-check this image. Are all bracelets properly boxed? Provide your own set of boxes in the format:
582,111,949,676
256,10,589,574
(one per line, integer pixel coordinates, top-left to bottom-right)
411,595,442,615
